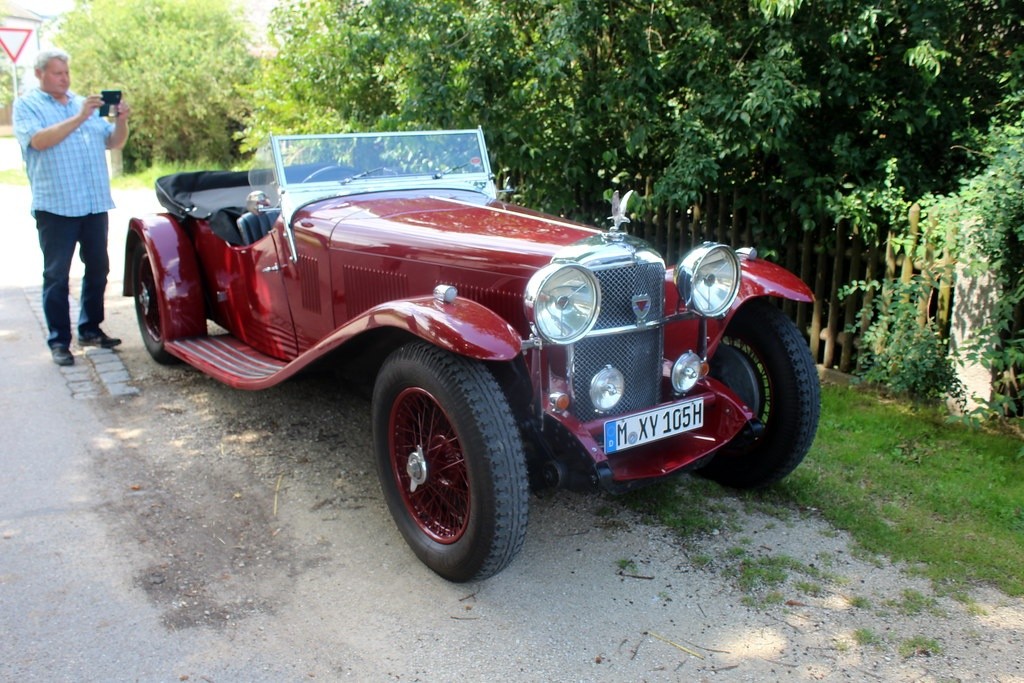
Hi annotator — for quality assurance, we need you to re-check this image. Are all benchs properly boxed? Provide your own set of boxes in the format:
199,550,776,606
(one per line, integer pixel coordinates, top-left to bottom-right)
155,162,362,246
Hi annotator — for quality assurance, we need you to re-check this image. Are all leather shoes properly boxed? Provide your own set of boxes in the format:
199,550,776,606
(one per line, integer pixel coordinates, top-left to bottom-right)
52,346,74,366
78,330,121,348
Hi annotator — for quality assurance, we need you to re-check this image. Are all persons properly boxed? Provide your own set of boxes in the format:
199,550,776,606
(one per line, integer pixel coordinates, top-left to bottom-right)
12,50,129,367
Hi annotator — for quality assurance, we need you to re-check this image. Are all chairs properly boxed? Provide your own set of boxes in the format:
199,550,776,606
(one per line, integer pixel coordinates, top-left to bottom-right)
236,207,280,248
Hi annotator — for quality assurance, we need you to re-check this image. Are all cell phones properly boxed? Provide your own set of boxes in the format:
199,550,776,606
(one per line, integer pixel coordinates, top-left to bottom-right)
101,90,122,105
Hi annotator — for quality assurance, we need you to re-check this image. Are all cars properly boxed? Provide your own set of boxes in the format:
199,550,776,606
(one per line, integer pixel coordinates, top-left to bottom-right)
122,125,821,582
